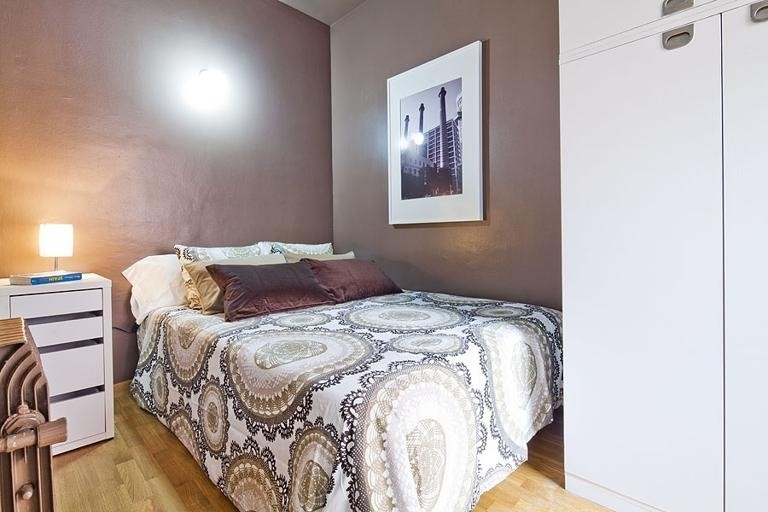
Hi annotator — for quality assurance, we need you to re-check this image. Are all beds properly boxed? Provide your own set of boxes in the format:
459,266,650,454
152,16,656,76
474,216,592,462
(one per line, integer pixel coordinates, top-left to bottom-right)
120,253,563,512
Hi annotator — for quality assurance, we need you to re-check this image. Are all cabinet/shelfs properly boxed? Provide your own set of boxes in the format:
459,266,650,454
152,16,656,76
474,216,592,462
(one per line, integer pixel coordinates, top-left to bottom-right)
556,0,768,511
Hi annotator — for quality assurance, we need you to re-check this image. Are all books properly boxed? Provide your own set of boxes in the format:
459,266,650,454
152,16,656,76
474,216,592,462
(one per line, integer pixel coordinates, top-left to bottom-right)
7,269,83,287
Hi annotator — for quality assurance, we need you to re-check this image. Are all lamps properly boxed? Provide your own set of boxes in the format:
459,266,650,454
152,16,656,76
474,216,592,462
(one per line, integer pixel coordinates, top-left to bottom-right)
36,222,74,270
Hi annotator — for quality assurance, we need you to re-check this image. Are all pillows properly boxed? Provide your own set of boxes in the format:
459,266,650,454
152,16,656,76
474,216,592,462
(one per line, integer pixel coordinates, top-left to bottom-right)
182,250,286,316
297,251,406,306
260,239,335,254
287,249,356,265
173,239,262,313
203,256,336,322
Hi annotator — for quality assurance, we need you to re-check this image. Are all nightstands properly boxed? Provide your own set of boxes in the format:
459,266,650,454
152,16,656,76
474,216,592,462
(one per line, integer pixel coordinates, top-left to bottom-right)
0,272,114,458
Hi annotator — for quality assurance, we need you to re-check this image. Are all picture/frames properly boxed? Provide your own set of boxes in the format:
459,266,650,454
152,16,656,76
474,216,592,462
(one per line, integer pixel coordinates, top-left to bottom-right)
386,40,486,226
